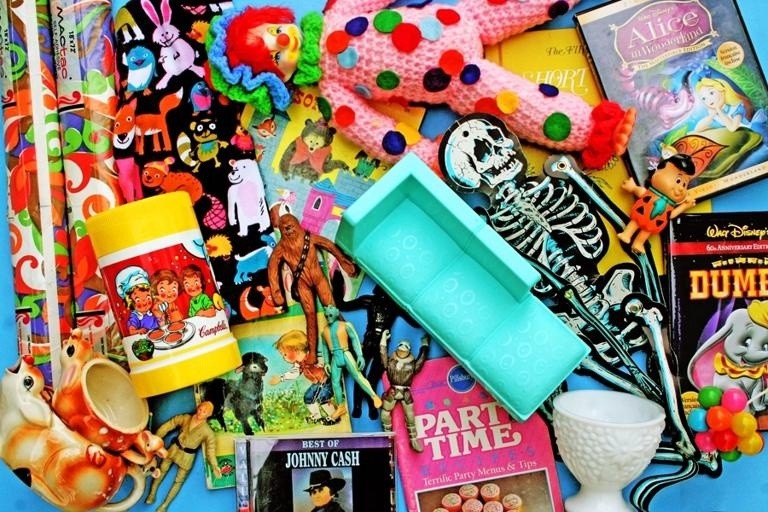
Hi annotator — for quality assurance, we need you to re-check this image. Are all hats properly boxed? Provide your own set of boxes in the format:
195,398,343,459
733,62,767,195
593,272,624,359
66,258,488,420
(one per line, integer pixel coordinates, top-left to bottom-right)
304,470,345,493
115,267,150,298
